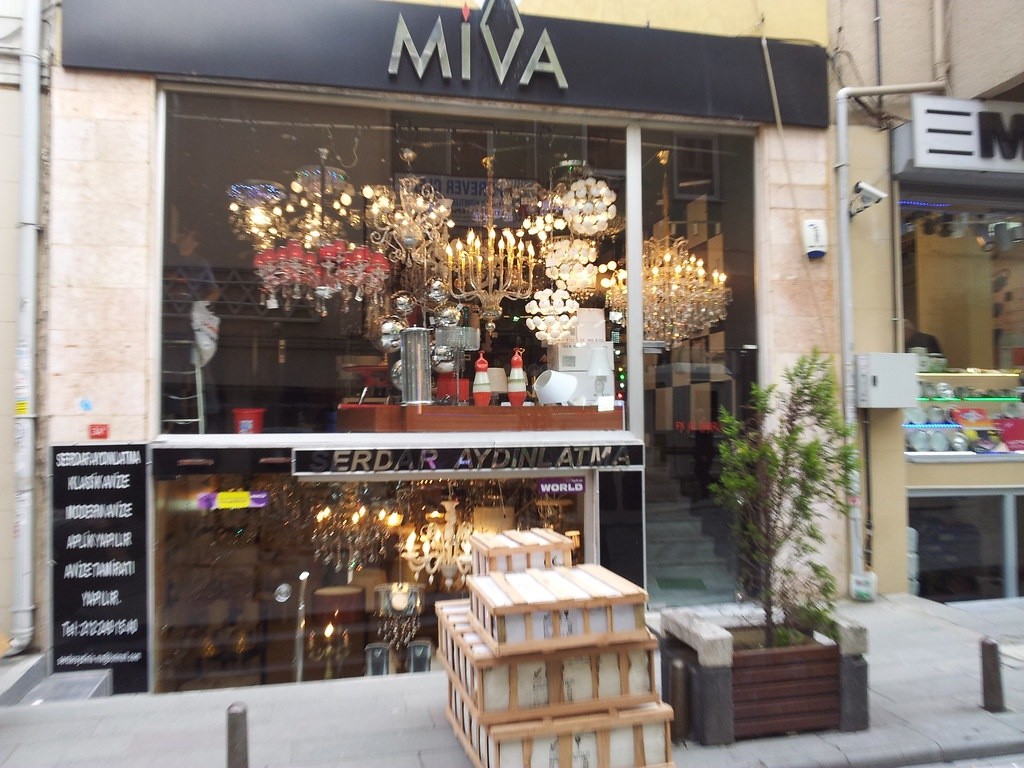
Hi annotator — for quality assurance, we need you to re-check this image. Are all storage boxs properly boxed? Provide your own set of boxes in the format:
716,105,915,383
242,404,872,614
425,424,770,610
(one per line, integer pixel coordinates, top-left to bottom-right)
434,526,675,768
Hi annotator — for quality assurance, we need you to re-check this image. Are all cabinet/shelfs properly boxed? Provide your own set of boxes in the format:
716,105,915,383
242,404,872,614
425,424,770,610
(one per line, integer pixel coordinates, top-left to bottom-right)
903,371,1024,486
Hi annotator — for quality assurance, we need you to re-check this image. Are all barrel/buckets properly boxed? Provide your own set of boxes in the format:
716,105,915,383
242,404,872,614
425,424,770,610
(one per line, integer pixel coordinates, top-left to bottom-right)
231,408,265,434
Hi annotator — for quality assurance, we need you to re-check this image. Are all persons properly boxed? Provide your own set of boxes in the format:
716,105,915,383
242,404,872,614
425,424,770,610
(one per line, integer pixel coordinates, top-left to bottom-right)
160,223,221,433
902,318,943,358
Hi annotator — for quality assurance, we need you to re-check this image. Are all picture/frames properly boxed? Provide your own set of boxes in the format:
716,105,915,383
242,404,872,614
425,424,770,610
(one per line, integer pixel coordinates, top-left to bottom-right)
651,191,729,435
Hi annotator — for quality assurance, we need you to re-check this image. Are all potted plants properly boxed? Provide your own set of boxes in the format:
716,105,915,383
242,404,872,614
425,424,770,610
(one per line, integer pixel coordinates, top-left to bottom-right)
658,347,872,749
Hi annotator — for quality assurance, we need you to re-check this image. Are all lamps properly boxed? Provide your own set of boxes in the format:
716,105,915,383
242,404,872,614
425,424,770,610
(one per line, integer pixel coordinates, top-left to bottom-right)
225,130,732,349
252,477,500,651
923,213,1024,253
